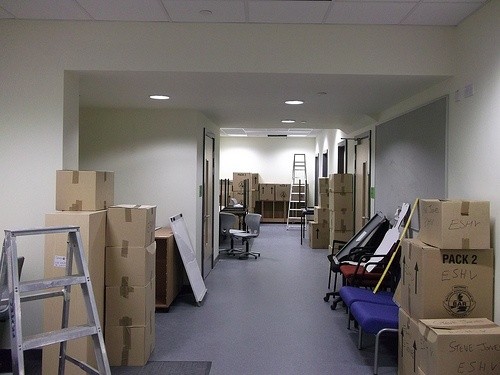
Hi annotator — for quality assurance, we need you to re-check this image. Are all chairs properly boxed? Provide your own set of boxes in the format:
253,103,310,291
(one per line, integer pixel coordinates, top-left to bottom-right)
324,240,402,327
219,212,244,257
230,213,263,260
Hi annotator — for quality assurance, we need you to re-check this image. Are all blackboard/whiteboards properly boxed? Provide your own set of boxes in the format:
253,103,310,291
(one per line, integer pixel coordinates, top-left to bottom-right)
169,213,209,307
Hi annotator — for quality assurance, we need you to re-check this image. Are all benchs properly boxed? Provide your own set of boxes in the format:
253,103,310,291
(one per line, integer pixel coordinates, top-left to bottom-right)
339,261,399,375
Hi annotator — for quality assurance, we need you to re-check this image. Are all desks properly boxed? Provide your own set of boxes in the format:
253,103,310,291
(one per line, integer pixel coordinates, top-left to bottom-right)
220,178,250,211
300,209,315,246
154,225,181,308
297,179,315,212
220,209,249,246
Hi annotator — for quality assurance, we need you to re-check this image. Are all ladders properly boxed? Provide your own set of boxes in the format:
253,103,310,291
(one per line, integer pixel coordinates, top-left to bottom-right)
285,153,308,230
3,223,112,374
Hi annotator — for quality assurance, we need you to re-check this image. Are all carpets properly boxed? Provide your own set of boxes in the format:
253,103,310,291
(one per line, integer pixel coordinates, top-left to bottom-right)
0,360,213,375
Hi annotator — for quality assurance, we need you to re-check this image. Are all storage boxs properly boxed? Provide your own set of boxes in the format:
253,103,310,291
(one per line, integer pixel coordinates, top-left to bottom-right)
42,169,158,375
219,172,309,229
391,199,500,375
308,174,356,254
